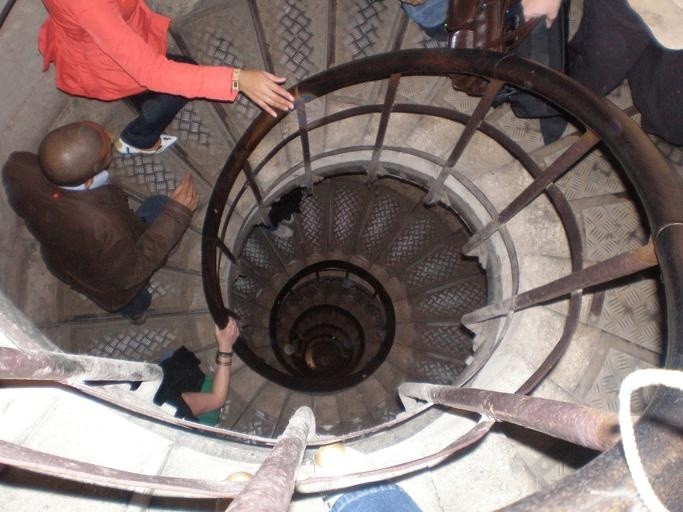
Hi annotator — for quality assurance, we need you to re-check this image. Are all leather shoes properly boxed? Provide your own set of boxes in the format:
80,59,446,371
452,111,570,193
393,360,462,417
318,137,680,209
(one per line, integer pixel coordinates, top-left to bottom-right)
115,134,178,155
540,116,567,145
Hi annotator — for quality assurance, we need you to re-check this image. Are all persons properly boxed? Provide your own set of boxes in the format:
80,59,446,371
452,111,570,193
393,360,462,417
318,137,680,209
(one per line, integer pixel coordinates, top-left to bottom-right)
1,119,203,327
506,1,682,151
133,314,240,429
33,1,296,158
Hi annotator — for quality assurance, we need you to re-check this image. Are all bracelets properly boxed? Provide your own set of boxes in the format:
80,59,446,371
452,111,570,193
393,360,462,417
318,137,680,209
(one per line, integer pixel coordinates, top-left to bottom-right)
217,350,234,359
231,67,241,93
214,359,232,367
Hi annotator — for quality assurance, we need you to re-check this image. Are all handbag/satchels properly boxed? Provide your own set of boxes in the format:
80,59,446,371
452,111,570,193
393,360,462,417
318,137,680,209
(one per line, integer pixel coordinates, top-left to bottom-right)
446,0,568,117
